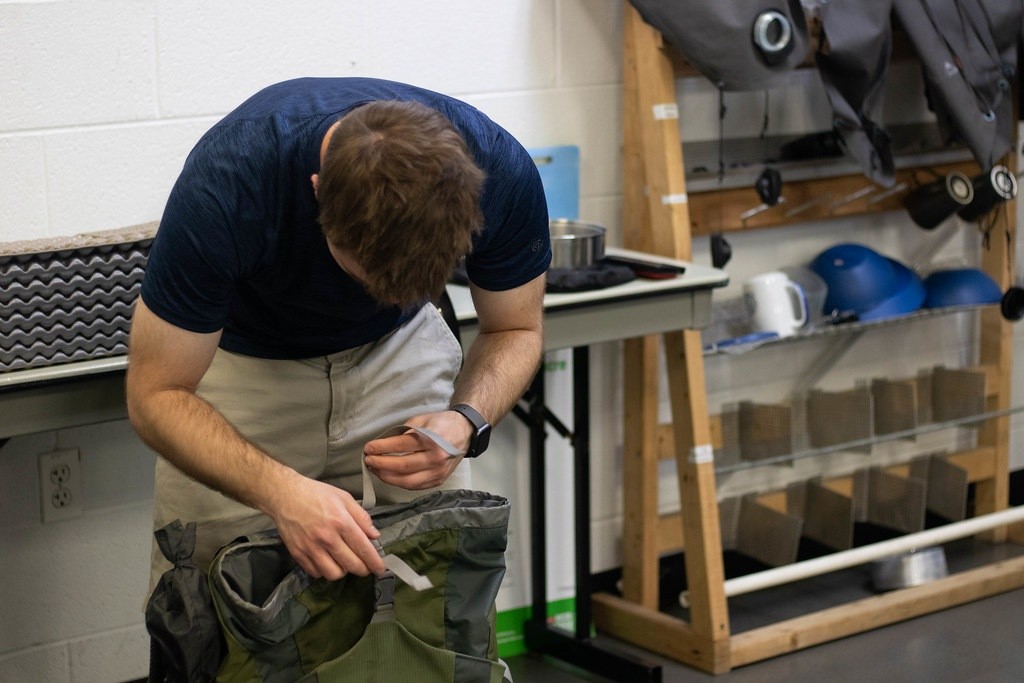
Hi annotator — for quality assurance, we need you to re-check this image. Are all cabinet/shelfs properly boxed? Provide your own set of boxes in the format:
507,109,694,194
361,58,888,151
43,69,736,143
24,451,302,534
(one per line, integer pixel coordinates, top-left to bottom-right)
590,0,1024,678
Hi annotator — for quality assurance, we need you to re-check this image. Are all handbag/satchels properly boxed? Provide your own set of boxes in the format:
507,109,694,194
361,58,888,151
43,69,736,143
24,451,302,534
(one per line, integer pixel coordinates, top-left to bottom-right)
144,426,513,683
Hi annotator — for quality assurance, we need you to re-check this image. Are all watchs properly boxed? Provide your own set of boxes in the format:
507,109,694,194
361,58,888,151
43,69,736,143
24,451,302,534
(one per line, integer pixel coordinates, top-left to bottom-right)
451,403,492,460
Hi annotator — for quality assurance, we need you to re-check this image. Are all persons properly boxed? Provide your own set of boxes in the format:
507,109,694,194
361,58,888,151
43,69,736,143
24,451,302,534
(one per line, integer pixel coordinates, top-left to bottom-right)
127,77,553,683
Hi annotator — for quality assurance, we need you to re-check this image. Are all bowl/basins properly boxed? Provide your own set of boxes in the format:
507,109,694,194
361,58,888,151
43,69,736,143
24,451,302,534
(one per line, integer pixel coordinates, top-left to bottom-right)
550,221,607,271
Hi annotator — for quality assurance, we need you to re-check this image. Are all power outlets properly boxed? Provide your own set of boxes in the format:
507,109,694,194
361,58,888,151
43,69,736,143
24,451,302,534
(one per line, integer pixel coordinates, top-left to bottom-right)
40,449,84,524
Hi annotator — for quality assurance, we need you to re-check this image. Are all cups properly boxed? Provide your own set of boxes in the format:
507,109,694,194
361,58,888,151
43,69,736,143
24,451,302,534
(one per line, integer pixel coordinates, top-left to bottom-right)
743,272,810,337
